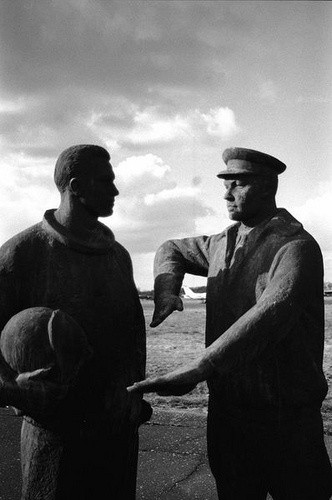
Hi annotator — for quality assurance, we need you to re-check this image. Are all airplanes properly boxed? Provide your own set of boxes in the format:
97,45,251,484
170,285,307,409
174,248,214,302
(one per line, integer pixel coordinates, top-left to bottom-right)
181,284,206,304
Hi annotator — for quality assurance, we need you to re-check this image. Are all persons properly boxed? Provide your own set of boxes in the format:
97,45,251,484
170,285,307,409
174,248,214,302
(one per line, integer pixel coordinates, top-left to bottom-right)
0,145,154,500
144,147,331,500
